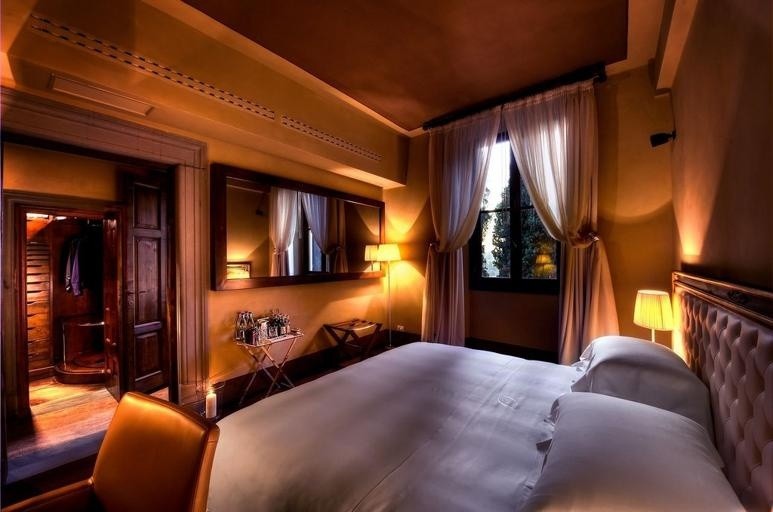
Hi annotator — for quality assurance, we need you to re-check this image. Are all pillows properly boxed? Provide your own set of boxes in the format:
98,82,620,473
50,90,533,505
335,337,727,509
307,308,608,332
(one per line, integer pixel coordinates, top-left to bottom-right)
573,331,711,428
530,392,745,511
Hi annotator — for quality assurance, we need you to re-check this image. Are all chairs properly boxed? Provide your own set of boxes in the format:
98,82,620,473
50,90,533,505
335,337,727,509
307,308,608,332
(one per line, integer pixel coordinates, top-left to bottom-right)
1,392,219,512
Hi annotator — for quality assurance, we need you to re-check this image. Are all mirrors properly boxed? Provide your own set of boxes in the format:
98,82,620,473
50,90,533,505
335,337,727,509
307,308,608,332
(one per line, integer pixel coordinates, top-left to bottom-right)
210,161,385,292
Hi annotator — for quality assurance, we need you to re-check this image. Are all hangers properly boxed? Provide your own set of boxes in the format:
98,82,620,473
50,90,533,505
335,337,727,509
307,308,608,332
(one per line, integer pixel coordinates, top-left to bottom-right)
68,215,104,230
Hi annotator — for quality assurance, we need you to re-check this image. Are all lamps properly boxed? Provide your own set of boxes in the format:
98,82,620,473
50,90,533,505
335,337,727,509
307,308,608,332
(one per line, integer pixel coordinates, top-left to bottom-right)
378,245,400,345
633,289,673,342
365,243,378,265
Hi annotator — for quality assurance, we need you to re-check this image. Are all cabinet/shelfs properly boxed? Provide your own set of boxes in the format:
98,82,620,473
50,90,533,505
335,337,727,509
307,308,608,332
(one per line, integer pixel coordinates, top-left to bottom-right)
236,332,304,402
51,215,108,383
324,318,381,360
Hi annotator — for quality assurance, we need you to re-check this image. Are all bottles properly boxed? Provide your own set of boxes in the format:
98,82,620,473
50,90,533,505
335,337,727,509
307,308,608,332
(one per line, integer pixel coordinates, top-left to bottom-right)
236,310,292,346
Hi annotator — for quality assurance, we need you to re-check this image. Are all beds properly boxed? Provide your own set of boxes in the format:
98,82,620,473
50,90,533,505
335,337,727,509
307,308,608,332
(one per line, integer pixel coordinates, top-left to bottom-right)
206,269,772,511
